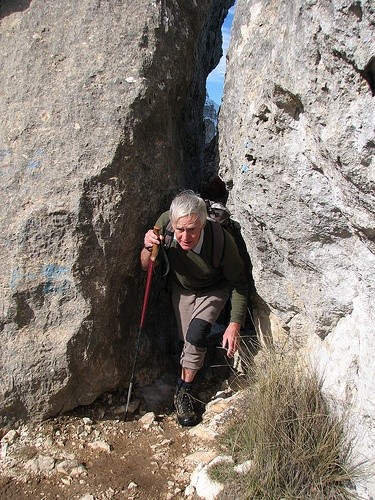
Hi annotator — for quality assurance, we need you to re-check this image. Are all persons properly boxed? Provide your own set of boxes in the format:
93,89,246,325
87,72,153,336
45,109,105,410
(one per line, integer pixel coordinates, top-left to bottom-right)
142,189,248,427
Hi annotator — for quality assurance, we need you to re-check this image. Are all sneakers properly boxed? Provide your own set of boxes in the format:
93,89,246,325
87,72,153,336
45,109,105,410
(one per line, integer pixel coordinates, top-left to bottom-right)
171,384,205,427
